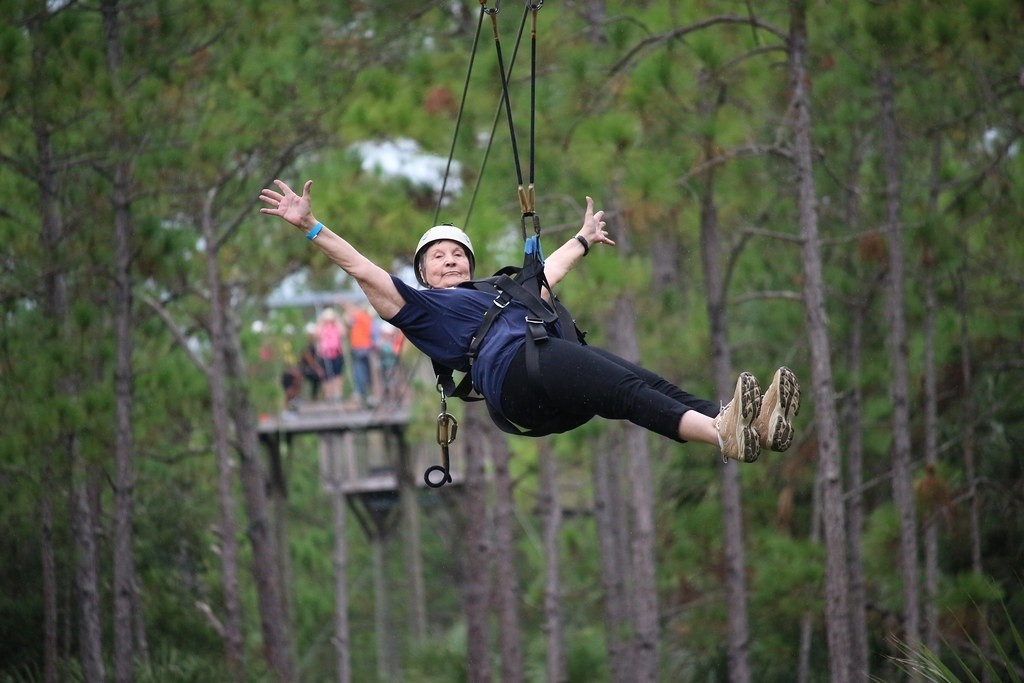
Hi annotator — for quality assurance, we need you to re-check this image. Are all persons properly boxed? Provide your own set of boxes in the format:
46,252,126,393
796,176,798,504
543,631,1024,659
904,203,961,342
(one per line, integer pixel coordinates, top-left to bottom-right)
252,296,408,416
260,180,801,463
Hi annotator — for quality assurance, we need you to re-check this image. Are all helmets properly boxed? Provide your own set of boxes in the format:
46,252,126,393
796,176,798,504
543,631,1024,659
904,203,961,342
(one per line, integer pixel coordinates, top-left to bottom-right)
414,225,475,289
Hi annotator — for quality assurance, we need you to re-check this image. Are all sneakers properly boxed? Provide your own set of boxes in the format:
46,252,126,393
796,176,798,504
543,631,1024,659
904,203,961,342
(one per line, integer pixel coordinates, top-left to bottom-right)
755,367,801,452
712,372,762,464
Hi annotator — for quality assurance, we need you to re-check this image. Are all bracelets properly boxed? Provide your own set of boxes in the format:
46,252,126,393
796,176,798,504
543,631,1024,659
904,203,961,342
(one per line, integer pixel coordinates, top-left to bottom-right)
306,222,323,241
571,235,589,256
